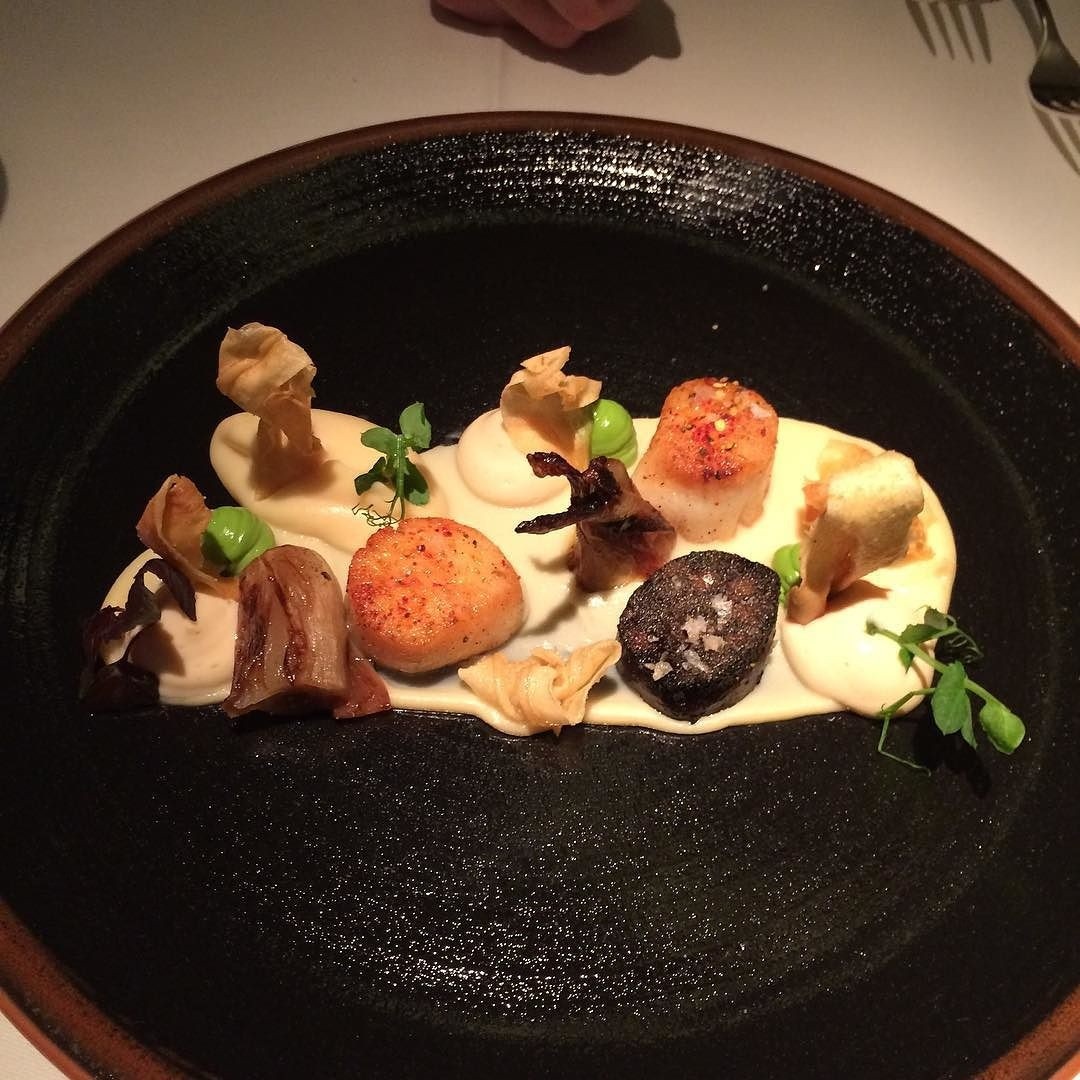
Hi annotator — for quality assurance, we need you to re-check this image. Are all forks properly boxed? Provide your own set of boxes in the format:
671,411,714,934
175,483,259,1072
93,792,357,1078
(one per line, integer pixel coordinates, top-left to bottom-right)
1026,0,1080,114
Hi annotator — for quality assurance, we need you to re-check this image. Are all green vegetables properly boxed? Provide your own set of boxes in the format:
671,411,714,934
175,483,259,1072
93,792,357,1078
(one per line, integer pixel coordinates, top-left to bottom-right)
350,401,434,530
865,609,1027,773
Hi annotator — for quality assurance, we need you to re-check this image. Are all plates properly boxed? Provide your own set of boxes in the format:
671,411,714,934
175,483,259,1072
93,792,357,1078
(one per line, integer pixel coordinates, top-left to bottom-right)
0,111,1080,1077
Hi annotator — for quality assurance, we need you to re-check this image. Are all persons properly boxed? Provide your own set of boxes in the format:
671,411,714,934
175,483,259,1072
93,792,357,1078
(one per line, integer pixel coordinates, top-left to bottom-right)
436,0,638,48
